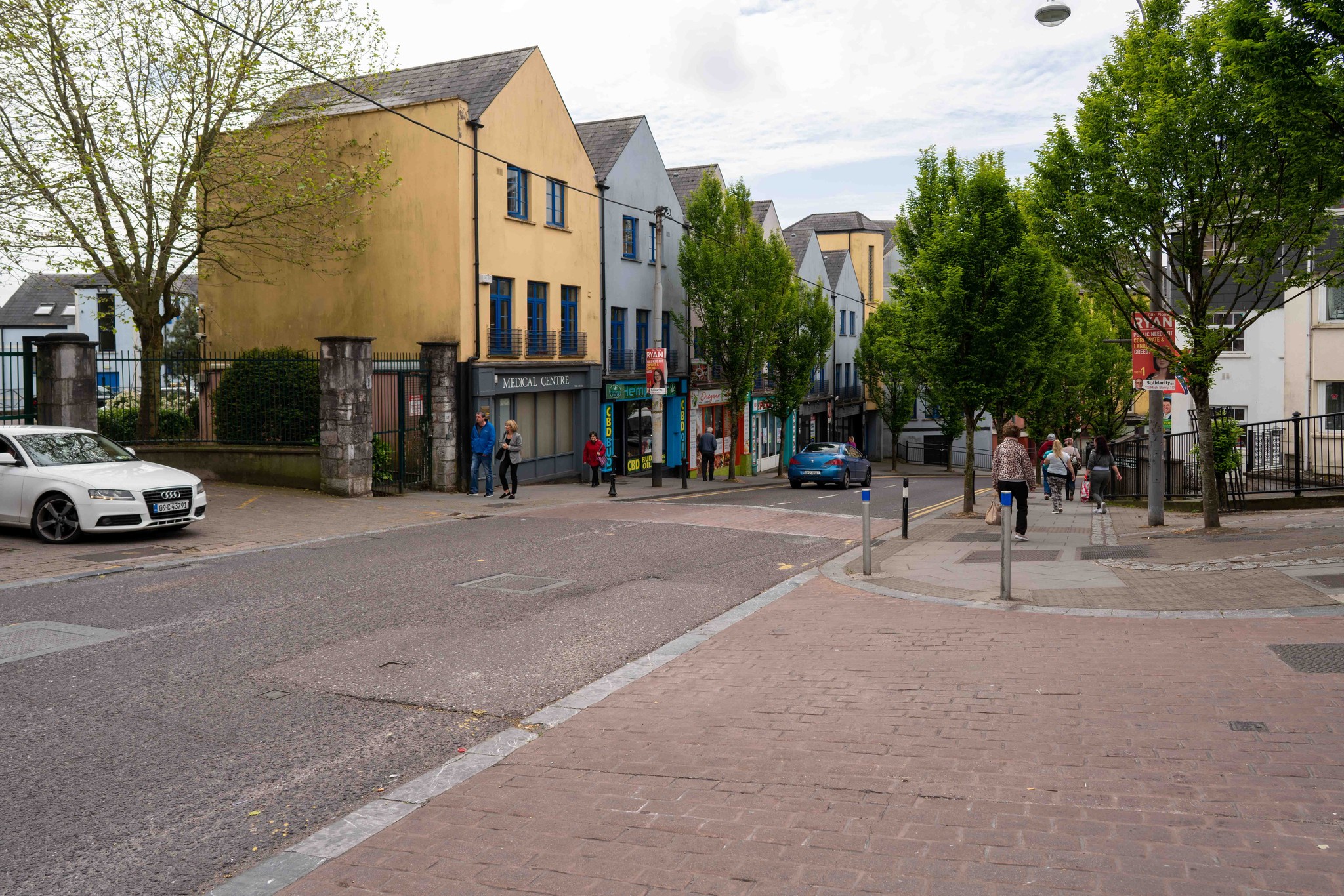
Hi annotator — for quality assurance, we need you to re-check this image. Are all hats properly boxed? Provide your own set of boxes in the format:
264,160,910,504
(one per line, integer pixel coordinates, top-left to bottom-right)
1047,433,1056,441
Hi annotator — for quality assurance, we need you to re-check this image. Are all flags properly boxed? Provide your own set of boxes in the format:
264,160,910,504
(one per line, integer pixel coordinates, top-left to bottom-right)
1163,349,1190,394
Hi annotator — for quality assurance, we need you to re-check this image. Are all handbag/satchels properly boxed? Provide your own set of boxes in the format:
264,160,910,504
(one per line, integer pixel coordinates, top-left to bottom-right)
985,489,1001,525
597,443,608,468
1070,448,1082,470
1081,475,1091,500
496,447,504,460
1067,467,1071,474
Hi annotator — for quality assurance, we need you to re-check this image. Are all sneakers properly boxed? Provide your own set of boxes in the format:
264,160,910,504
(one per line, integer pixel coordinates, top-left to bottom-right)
1093,508,1102,513
484,493,493,497
1101,502,1107,514
467,491,478,495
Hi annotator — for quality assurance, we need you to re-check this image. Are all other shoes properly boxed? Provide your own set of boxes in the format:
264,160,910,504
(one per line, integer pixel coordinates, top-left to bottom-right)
703,478,707,481
1044,494,1049,500
1052,510,1059,514
508,495,515,499
709,478,715,481
1015,532,1029,540
499,490,510,499
1066,496,1069,500
1049,496,1052,499
1070,496,1074,501
1058,506,1063,513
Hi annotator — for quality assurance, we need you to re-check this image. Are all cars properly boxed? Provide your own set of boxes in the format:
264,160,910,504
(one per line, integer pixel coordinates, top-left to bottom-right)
30,385,115,420
0,423,206,543
786,441,873,491
139,383,200,406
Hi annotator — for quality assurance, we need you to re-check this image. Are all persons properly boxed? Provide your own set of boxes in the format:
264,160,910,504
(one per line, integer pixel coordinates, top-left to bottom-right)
1133,378,1146,390
698,427,718,481
499,419,522,499
1085,435,1122,515
467,412,496,497
647,387,651,394
847,436,856,448
583,431,606,487
1037,433,1081,514
1146,347,1175,380
1162,398,1171,420
651,369,665,388
991,421,1036,540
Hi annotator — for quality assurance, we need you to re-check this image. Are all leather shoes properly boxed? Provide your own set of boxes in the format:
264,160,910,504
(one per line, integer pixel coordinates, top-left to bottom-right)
592,483,595,487
595,482,599,486
